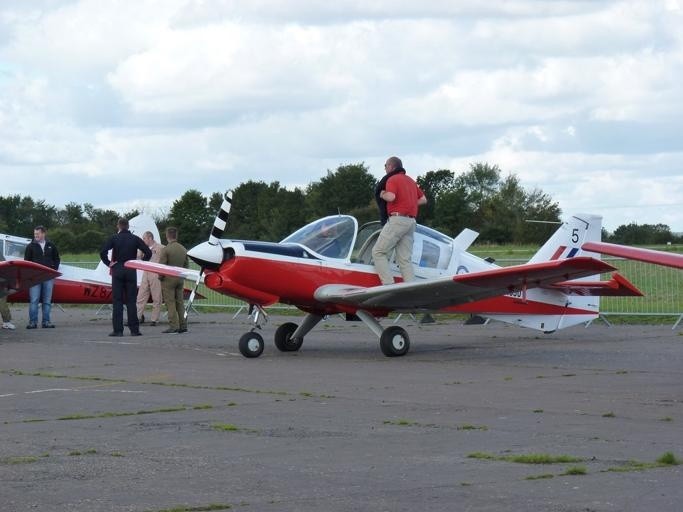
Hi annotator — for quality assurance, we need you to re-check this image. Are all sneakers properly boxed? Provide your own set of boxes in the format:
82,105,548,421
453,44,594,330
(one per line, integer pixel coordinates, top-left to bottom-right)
2,321,16,331
109,332,123,336
161,327,179,333
26,324,38,330
42,325,55,328
131,332,142,336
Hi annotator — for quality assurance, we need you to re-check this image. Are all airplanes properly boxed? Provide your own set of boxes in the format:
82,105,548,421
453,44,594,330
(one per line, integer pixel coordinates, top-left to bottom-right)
118,188,648,360
0,213,209,316
579,240,682,274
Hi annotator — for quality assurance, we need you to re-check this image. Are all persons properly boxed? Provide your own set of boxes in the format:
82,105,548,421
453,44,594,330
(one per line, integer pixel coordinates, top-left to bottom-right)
0,246,16,329
372,157,429,285
158,227,190,333
301,217,342,258
24,225,61,328
136,231,167,326
99,217,152,337
